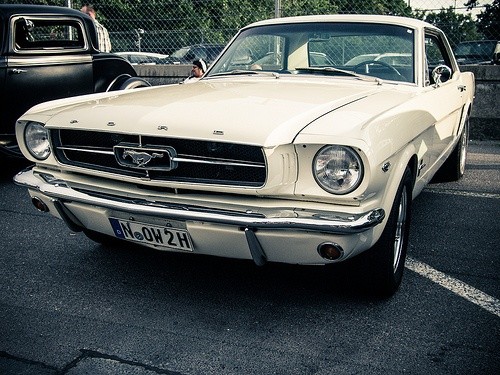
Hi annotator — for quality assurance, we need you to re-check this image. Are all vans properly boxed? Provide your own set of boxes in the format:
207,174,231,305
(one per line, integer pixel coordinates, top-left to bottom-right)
159,44,269,64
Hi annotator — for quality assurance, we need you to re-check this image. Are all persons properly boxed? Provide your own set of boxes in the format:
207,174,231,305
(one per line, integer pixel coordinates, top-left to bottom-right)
183,58,208,82
80,6,111,53
250,64,264,71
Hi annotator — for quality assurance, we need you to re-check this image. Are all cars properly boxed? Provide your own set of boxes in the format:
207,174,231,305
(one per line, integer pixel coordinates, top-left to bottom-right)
439,41,500,66
12,14,475,294
343,54,381,67
0,4,152,142
369,52,414,66
113,52,169,65
250,52,337,65
